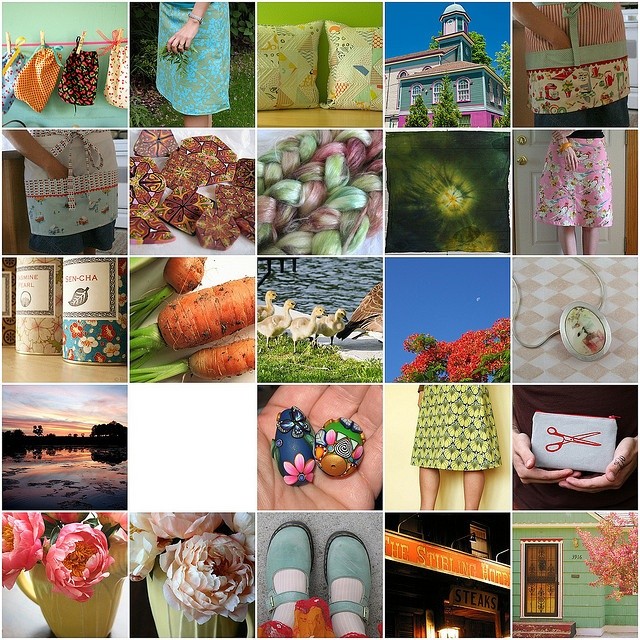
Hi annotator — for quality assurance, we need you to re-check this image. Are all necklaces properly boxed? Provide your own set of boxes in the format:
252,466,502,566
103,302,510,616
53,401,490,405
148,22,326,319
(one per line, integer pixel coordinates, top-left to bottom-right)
511,258,612,361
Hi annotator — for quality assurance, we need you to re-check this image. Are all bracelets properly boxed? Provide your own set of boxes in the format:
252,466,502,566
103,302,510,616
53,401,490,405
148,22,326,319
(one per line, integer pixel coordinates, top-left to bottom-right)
189,13,204,30
557,141,572,157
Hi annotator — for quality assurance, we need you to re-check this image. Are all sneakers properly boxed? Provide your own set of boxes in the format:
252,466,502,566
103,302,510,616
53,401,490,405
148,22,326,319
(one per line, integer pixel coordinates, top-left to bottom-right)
265,520,313,613
324,531,370,628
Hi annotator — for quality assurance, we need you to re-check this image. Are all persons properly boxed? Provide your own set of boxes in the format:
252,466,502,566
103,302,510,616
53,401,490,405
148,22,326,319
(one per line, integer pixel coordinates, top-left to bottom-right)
258,380,383,513
154,3,232,127
257,522,382,638
513,2,629,127
535,131,614,256
2,129,119,254
383,383,512,512
512,385,638,511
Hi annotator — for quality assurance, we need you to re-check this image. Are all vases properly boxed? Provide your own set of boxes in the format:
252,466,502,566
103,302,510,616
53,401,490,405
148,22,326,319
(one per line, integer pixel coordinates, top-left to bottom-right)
15,533,128,638
146,558,255,638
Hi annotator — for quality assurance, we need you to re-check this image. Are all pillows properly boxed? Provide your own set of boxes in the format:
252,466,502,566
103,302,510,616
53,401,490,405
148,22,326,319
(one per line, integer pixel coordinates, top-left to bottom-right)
257,20,324,111
321,19,383,111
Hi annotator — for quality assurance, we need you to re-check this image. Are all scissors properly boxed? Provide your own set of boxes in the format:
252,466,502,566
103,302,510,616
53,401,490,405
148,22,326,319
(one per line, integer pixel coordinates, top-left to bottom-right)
546,427,601,452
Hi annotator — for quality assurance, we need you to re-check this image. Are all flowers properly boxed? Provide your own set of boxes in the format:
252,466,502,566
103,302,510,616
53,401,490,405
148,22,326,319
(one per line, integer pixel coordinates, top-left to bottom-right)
2,512,128,604
130,512,255,626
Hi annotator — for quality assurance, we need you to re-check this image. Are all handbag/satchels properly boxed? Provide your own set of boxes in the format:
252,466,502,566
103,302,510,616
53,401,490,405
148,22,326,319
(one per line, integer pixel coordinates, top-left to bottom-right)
528,411,621,473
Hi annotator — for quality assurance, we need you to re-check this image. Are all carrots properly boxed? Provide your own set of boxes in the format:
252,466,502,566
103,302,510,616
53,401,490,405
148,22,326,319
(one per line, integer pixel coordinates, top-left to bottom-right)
126,277,256,368
131,257,207,326
129,339,256,383
